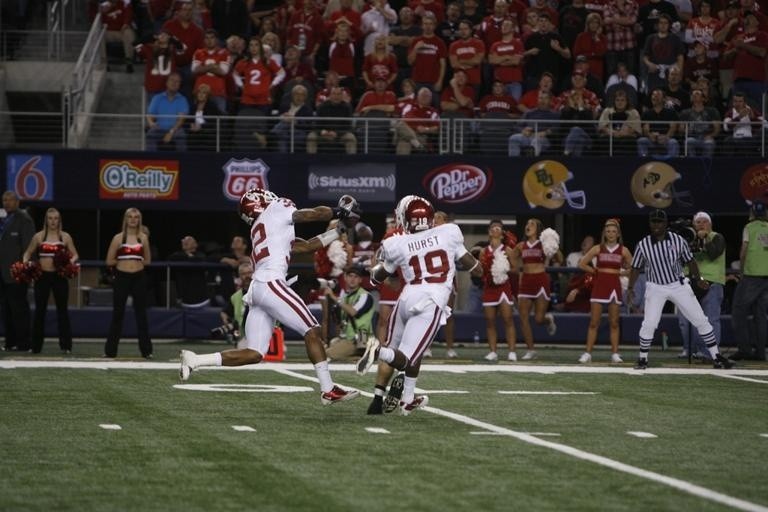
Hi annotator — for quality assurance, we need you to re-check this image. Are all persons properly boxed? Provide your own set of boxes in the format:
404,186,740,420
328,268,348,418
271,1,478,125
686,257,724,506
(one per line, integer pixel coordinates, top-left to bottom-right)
2,189,768,416
89,1,768,157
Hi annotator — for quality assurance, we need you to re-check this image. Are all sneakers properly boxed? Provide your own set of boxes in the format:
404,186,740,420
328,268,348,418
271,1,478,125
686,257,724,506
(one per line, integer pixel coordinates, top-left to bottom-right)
381,373,404,414
484,351,498,361
398,394,429,417
0,344,155,359
578,352,592,363
423,350,431,357
320,384,360,408
367,398,383,415
710,353,737,369
178,349,198,383
508,351,518,361
355,336,382,376
447,350,458,359
545,312,557,337
632,356,650,370
521,351,538,360
611,352,623,363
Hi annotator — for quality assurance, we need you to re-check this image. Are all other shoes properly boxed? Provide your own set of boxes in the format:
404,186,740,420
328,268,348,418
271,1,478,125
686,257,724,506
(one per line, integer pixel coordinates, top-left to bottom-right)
693,352,705,359
678,351,689,358
745,352,764,360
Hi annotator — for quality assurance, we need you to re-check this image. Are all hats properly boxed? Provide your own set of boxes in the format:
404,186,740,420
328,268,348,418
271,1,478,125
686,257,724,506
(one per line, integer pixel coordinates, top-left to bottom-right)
648,207,667,222
744,198,767,219
345,266,363,278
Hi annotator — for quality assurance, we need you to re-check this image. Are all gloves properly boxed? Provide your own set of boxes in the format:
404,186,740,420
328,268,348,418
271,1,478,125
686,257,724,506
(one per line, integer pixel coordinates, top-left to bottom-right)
336,220,349,235
339,200,363,221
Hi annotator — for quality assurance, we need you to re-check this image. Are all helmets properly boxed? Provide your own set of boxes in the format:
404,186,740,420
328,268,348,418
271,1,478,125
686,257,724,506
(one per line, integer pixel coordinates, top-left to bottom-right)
394,194,435,234
237,187,269,226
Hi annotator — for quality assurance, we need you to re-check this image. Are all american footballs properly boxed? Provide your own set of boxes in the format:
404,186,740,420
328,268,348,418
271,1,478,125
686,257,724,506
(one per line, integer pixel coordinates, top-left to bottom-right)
343,200,361,228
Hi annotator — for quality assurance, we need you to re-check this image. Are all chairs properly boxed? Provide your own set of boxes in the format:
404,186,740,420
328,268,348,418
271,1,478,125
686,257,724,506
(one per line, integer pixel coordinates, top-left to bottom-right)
68,1,768,157
